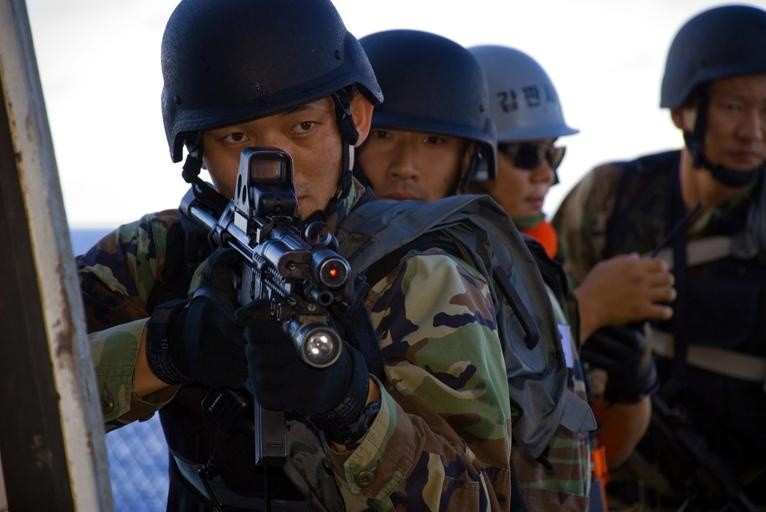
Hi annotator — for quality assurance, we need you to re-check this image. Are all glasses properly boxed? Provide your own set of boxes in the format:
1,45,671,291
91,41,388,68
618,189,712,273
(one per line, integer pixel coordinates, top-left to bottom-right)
498,142,567,172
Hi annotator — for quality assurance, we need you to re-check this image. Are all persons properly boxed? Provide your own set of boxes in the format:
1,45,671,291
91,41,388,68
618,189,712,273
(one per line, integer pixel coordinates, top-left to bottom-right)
74,0,513,510
463,43,681,509
544,3,765,507
338,24,602,509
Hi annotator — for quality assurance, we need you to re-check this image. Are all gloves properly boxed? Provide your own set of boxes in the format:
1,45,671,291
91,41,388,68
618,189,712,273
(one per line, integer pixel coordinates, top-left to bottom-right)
580,319,662,406
144,293,248,393
233,297,370,434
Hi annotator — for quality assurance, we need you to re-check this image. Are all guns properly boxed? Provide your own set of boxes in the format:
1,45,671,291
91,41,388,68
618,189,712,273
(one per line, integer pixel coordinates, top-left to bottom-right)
179,146,351,465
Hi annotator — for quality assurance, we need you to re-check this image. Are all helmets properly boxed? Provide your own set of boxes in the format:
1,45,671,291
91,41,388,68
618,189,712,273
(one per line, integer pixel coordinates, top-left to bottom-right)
161,0,385,164
357,30,499,181
660,5,766,108
465,46,579,143
